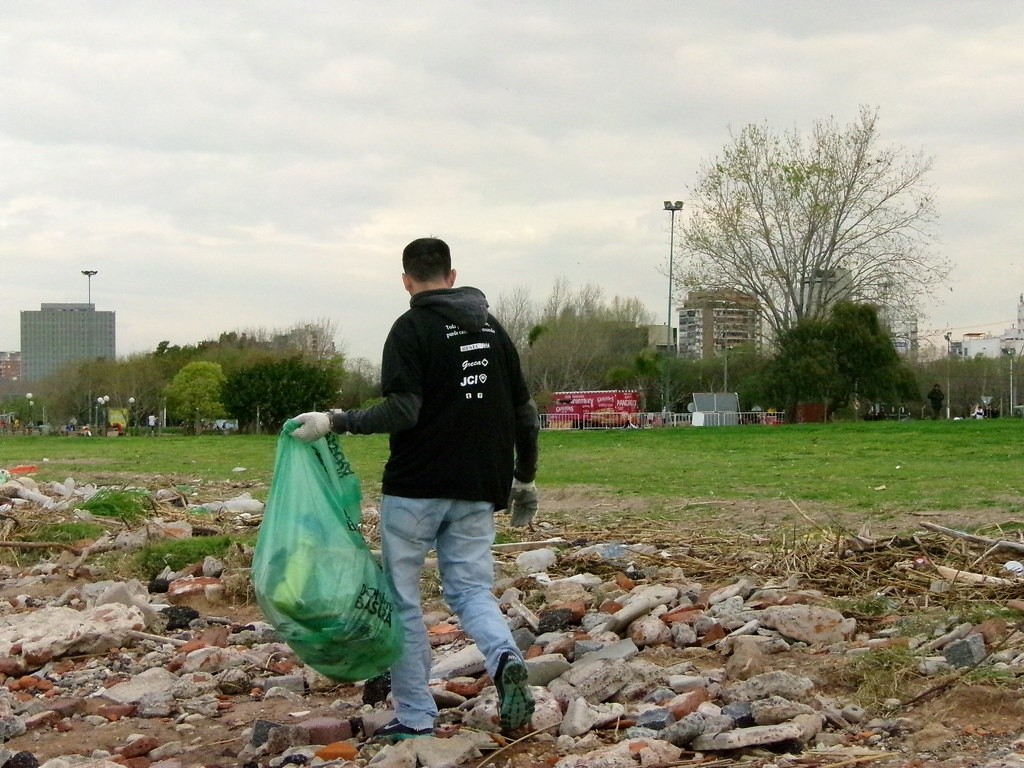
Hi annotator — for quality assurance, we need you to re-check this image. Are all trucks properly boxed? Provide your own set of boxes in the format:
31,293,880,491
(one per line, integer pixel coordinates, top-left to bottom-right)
194,418,238,431
545,390,640,426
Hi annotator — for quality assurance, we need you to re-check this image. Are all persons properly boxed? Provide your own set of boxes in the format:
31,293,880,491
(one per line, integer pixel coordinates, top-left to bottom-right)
927,384,945,420
969,406,984,419
0,415,124,436
289,238,540,737
148,413,158,436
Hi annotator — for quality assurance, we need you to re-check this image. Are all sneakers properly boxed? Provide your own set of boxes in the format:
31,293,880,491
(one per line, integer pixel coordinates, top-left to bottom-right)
371,717,435,739
493,651,535,730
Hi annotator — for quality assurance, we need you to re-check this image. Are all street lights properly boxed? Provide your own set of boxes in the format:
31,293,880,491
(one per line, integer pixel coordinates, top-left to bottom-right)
944,332,952,419
97,395,109,437
663,200,684,428
26,393,34,435
723,345,734,392
1002,348,1016,416
81,270,97,306
129,398,135,437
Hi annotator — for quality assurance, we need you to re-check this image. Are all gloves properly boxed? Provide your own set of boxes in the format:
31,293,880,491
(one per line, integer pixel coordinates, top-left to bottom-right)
503,478,539,528
284,409,342,442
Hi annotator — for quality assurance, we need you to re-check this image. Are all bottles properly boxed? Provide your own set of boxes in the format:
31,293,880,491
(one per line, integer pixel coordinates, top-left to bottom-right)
296,596,318,610
273,540,315,612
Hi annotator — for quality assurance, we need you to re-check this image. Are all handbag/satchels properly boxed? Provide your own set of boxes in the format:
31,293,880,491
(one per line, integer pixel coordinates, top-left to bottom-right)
250,419,404,683
